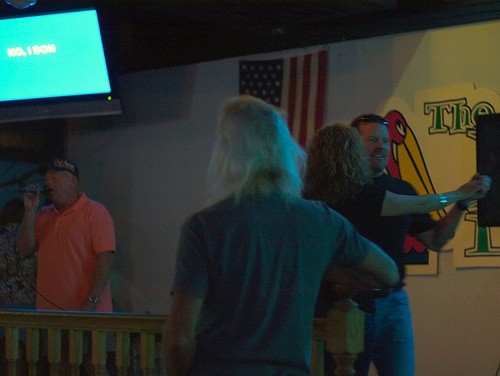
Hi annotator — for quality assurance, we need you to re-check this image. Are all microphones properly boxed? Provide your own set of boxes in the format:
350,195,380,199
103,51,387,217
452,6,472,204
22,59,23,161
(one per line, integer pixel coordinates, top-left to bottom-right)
16,188,49,193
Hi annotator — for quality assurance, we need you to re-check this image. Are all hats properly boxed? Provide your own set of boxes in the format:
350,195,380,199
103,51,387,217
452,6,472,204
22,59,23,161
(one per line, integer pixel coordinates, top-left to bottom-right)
37,158,79,181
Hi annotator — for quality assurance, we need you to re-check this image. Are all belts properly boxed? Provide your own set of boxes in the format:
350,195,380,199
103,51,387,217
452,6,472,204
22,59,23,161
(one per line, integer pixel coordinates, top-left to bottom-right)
368,284,403,299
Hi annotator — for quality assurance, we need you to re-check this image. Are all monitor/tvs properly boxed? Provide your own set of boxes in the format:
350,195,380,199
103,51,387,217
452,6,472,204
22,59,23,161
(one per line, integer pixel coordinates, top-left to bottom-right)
0,5,124,124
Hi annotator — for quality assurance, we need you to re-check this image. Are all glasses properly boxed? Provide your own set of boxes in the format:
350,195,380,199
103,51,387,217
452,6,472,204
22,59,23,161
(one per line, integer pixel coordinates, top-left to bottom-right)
357,114,389,125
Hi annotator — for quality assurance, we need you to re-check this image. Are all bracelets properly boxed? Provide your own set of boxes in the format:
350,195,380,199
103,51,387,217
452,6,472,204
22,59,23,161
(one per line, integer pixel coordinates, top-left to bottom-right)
455,202,468,211
438,193,448,208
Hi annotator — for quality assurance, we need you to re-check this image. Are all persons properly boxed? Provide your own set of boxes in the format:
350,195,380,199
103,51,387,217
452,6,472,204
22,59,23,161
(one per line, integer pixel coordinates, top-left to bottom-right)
298,123,480,376
162,95,398,376
16,158,117,312
350,113,491,376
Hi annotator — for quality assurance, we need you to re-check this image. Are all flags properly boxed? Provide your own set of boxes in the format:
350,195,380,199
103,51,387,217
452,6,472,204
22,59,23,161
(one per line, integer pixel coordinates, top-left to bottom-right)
240,50,326,154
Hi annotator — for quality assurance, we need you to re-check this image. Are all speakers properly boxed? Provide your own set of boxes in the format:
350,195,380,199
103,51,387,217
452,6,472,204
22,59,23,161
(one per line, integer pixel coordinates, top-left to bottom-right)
475,113,500,227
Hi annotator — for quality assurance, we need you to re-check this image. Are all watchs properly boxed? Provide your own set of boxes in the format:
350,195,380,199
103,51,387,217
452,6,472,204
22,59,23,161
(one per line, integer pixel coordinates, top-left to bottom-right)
88,294,100,302
